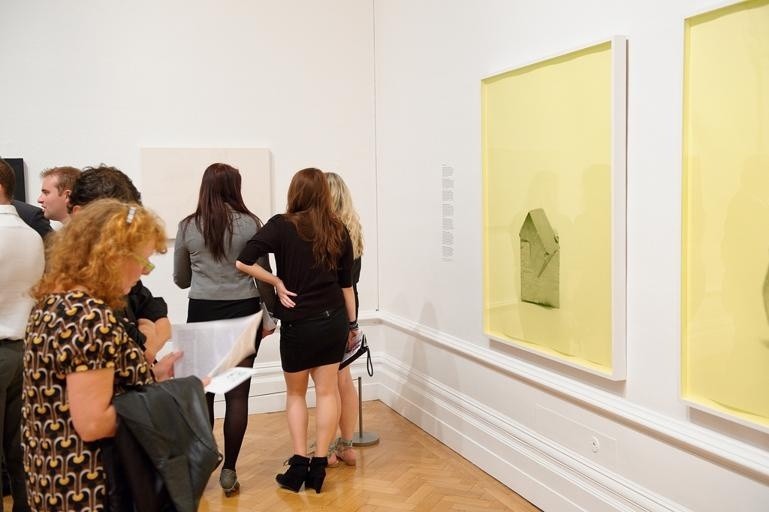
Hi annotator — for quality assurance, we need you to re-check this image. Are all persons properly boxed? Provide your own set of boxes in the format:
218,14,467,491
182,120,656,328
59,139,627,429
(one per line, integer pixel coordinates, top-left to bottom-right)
18,195,212,511
312,170,366,469
174,163,278,499
0,154,171,512
233,165,374,498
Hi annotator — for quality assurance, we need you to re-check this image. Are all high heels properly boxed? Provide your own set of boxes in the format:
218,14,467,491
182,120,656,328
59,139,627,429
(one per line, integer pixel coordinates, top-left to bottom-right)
218,469,240,498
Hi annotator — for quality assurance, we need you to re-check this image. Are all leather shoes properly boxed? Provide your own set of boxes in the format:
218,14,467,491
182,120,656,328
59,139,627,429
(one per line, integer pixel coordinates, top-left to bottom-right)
333,440,356,466
307,451,338,467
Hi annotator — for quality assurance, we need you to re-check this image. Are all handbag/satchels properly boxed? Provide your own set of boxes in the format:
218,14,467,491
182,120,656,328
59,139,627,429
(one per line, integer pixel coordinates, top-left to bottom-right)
258,300,276,338
337,334,373,378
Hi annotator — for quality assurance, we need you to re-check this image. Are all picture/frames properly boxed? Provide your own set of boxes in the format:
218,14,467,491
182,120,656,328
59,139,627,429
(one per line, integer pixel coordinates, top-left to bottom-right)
677,0,769,435
475,35,627,381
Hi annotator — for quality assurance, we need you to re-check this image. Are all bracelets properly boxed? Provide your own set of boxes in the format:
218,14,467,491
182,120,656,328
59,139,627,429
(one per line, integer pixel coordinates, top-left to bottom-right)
346,320,360,331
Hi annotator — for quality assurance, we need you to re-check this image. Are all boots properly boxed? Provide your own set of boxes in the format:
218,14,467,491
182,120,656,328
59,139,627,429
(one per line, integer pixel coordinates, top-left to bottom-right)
274,454,309,492
304,457,327,494
